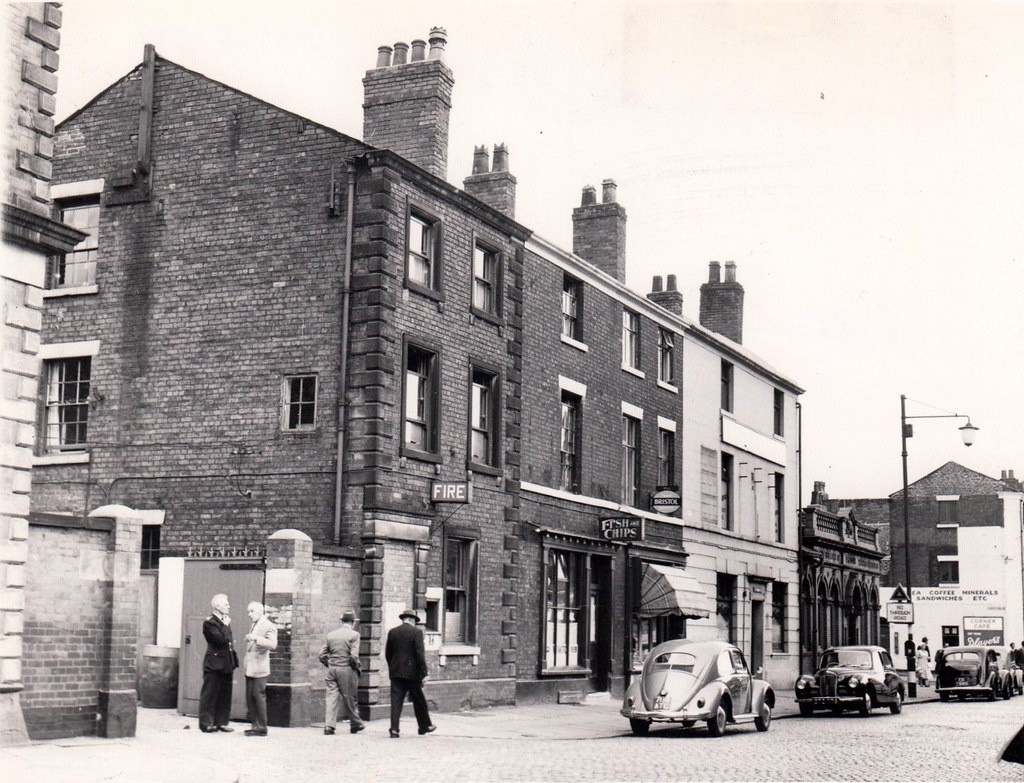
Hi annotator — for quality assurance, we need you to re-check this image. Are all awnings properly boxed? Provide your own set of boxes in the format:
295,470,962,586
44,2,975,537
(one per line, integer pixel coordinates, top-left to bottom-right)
636,562,710,619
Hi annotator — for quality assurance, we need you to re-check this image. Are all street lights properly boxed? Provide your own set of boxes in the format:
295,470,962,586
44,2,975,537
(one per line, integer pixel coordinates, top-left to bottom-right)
898,394,981,697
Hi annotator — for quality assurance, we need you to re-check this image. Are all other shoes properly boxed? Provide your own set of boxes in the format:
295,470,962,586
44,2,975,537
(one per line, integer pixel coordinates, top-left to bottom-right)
350,724,366,733
324,727,334,735
244,729,267,736
217,725,233,732
202,725,217,732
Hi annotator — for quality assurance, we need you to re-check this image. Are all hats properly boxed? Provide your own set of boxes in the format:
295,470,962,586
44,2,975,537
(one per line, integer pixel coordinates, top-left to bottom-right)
339,609,360,621
399,610,420,623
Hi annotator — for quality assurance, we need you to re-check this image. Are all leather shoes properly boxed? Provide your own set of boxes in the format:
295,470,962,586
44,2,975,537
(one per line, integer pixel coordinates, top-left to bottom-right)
418,725,436,735
391,730,399,738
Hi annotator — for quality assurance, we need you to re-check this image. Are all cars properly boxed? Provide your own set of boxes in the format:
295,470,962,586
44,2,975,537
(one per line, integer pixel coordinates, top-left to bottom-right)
618,639,778,738
794,646,906,718
934,647,1003,703
995,649,1024,699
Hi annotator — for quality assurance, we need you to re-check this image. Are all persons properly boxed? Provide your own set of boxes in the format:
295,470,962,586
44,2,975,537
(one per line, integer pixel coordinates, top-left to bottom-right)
319,610,366,735
199,594,239,733
1010,641,1024,682
243,602,278,736
916,637,930,687
935,643,949,689
385,609,437,738
